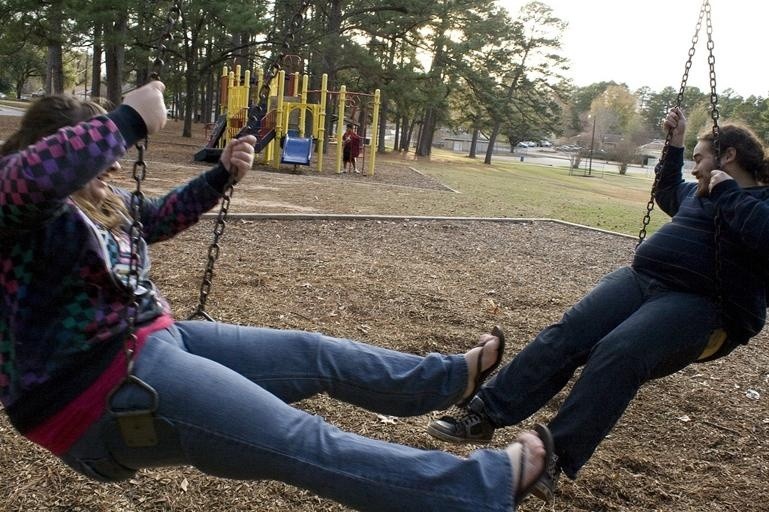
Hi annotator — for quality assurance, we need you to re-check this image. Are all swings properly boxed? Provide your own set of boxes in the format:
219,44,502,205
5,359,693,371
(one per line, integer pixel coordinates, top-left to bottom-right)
637,0,728,361
109,1,313,468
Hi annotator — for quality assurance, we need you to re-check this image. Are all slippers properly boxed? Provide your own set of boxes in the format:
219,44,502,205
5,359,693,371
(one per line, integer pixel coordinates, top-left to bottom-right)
512,420,553,511
456,322,506,410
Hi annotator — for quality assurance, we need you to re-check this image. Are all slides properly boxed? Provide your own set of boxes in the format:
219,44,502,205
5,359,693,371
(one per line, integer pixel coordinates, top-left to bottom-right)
280,134,312,166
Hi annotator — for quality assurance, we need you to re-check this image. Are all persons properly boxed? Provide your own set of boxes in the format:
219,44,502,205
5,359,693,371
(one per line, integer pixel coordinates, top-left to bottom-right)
2,80,553,512
425,105,768,504
342,123,361,174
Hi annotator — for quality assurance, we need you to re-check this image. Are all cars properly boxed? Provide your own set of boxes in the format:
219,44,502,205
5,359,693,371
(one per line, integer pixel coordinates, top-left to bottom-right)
517,140,580,152
32,88,45,97
167,109,176,117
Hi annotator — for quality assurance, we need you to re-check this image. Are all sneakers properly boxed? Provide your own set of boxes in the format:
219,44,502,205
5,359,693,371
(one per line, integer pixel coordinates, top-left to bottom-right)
532,452,561,501
341,168,360,173
427,395,496,445
